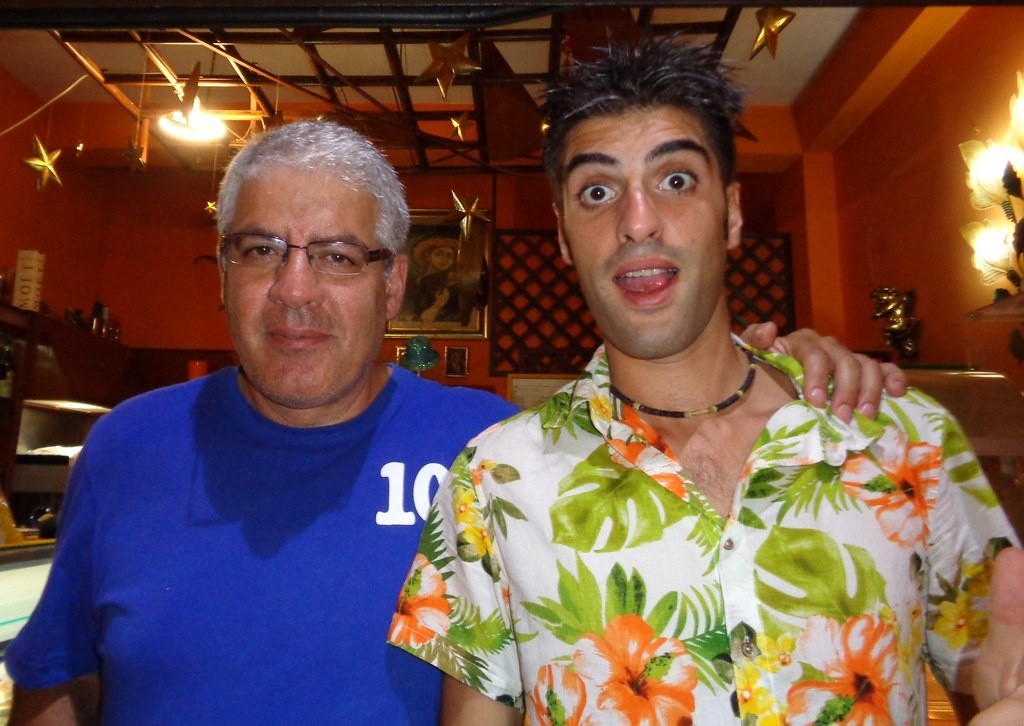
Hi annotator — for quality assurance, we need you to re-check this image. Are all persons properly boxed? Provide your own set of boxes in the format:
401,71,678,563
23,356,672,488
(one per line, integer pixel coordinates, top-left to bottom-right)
2,121,909,726
382,37,1023,725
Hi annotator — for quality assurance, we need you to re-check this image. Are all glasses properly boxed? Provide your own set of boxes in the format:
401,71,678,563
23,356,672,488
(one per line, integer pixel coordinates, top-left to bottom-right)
217,231,393,275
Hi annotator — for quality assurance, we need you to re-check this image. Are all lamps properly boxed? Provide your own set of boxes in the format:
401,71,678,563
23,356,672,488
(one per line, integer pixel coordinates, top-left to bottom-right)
159,96,228,144
961,71,1024,289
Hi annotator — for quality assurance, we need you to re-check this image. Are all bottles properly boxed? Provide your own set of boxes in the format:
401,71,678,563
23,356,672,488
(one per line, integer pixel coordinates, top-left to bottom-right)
88,302,102,336
1,330,14,398
100,305,109,338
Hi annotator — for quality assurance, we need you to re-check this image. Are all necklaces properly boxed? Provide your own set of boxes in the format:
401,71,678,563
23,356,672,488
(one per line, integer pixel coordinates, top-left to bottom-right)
608,342,760,417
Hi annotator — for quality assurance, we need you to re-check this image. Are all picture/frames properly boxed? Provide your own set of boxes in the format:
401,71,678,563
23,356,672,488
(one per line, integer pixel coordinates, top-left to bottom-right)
397,347,420,376
379,207,488,340
444,346,469,377
